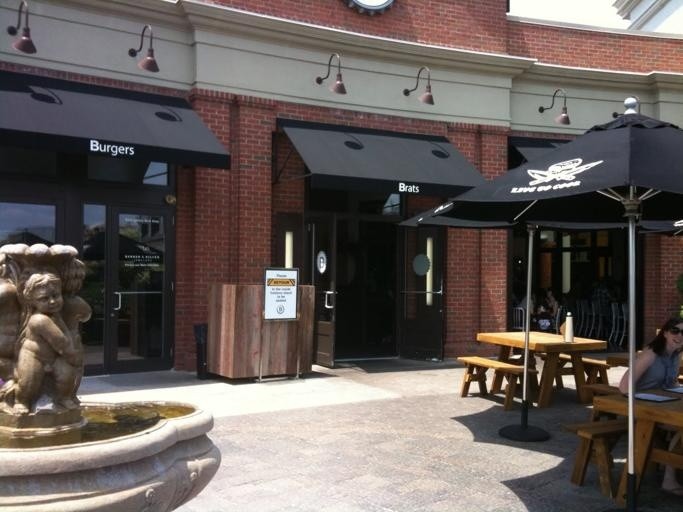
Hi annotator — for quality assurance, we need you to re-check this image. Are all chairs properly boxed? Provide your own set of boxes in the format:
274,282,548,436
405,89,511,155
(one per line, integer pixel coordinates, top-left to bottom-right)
569,297,629,347
511,307,525,331
546,305,562,334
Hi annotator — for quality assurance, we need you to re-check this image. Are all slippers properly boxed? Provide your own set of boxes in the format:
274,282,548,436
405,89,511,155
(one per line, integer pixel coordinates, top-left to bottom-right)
659,481,682,496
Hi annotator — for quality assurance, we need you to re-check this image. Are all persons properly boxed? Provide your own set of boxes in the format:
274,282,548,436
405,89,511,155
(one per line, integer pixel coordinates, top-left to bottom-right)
0,257,92,418
617,312,683,497
518,289,538,330
544,286,560,329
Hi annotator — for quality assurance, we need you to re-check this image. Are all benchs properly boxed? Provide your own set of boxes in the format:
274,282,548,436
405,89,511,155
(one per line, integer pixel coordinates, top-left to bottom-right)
457,356,539,412
535,351,611,391
562,418,628,498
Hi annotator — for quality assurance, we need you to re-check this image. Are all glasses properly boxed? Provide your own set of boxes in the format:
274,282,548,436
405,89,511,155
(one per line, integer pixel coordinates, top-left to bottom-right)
670,326,683,336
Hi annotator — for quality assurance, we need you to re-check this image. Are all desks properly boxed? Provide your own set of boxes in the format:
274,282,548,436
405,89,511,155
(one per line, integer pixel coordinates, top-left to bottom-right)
476,330,608,407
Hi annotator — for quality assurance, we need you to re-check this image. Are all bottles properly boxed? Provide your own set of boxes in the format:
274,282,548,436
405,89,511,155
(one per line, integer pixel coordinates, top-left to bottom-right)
564,312,574,343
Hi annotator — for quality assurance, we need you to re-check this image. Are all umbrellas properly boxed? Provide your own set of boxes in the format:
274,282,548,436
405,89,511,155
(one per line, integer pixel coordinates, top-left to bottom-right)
396,184,683,427
430,94,683,512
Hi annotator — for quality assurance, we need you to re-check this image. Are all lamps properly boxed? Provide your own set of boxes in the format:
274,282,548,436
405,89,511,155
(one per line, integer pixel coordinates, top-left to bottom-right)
314,51,347,95
128,23,160,72
6,0,38,53
404,65,434,105
538,88,570,125
